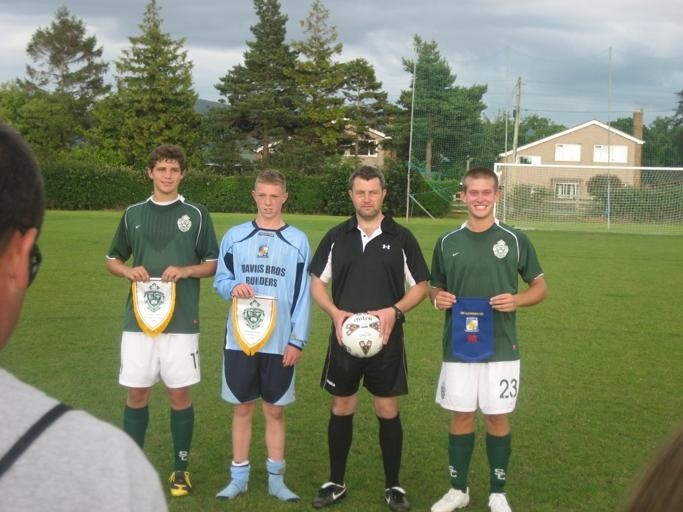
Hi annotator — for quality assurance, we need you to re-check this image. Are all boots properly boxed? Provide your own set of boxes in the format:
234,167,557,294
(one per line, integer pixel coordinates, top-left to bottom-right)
216,460,250,500
266,458,300,502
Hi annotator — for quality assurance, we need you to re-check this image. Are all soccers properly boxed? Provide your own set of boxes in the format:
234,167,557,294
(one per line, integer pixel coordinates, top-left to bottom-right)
341,313,383,359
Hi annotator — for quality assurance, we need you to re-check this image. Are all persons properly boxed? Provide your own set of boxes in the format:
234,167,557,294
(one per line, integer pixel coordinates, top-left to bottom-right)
105,144,218,498
214,168,314,503
431,167,547,512
0,122,169,512
307,165,429,504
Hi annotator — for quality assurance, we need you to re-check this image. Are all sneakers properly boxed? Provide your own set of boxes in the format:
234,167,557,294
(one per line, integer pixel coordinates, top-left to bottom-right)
488,492,512,512
313,481,346,508
384,486,410,512
431,487,469,512
169,471,192,497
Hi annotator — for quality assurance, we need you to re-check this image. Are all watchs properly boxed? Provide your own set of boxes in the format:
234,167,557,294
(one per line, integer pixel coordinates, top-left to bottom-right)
392,305,403,323
